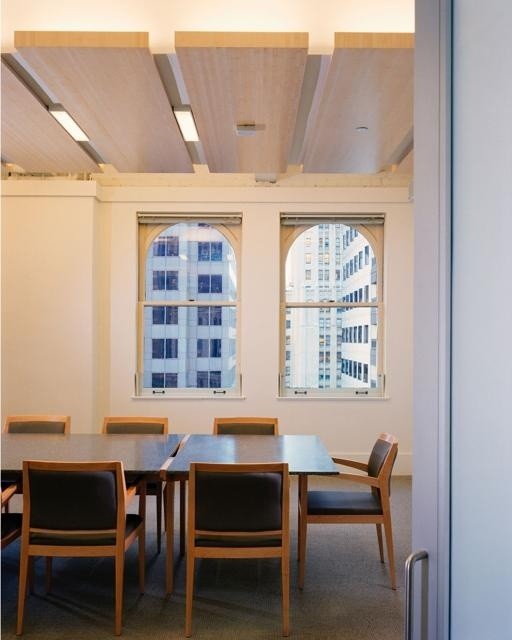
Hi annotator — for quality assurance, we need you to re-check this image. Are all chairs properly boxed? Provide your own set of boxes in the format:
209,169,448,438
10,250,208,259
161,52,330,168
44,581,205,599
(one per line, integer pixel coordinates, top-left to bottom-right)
3,414,72,438
1,485,55,601
298,432,399,592
213,417,279,436
15,457,146,637
102,414,170,555
183,460,294,640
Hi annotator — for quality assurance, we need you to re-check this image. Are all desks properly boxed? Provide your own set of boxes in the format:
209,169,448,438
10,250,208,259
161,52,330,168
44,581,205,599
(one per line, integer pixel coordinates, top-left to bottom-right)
2,428,340,599
